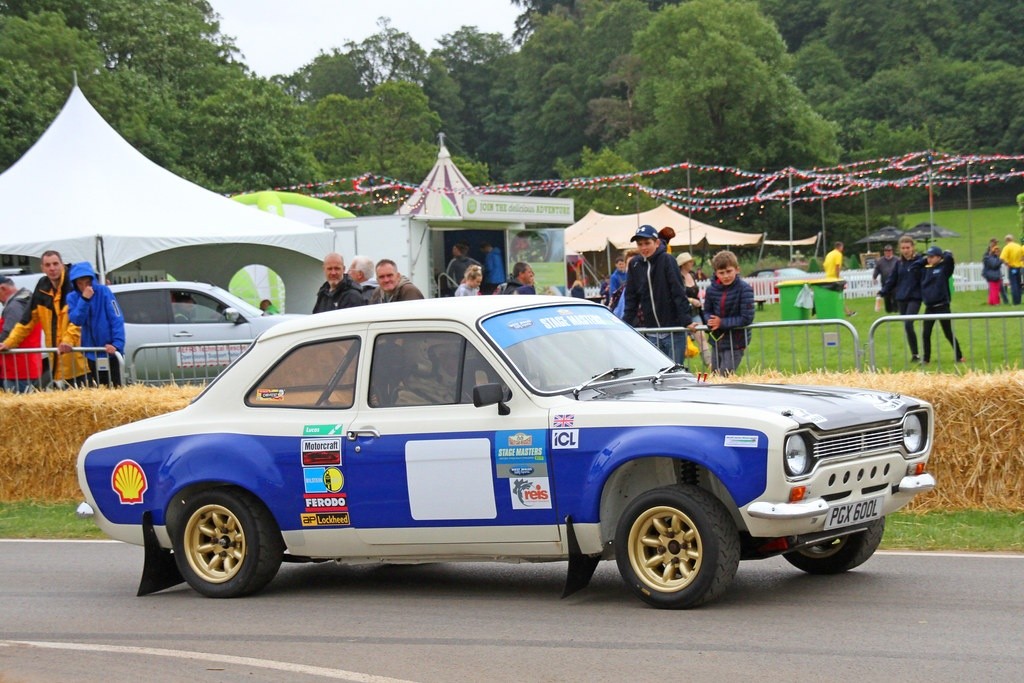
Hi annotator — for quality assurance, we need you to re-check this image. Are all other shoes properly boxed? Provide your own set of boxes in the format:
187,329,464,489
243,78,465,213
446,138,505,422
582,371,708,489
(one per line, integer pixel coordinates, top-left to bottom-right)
958,357,966,362
911,355,920,362
919,360,929,366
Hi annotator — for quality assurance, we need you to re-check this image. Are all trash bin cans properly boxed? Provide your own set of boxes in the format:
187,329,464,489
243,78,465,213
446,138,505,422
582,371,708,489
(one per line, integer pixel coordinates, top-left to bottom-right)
808,280,846,320
775,280,813,320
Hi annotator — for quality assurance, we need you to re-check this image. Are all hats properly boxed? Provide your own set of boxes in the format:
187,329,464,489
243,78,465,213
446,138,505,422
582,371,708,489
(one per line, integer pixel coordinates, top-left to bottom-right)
923,246,942,256
0,275,5,283
676,251,696,267
630,225,658,242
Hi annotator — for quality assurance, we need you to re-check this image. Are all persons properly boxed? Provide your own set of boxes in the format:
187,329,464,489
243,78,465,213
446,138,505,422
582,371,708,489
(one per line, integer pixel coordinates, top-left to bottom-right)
1,224,1024,407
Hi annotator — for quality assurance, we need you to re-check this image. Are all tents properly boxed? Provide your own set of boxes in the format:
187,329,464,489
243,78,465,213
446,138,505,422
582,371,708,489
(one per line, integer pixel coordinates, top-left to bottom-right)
564,201,822,281
0,72,337,289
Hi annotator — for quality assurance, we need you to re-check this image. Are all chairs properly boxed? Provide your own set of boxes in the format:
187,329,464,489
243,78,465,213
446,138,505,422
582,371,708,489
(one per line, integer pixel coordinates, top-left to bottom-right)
370,344,473,407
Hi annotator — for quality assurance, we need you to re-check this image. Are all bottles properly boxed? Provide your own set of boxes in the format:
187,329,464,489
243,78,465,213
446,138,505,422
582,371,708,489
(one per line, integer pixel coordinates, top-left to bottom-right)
875,294,881,312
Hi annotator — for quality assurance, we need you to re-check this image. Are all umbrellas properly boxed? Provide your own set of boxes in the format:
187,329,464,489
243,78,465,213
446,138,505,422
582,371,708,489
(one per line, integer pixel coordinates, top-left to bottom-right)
894,221,961,254
852,224,905,252
